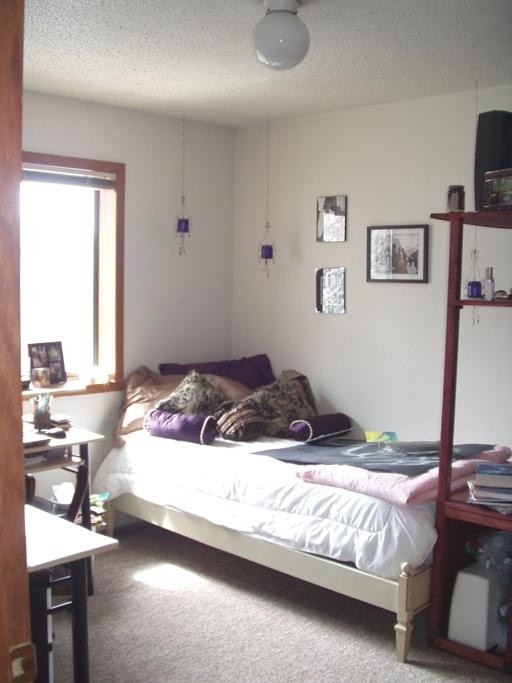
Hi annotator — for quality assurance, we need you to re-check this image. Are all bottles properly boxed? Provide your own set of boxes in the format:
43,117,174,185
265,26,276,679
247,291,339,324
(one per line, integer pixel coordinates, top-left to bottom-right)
466,249,496,302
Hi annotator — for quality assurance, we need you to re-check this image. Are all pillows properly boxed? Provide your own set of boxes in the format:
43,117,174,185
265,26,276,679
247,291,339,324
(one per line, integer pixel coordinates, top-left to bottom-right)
210,400,266,441
289,413,352,444
115,366,255,438
142,409,218,445
254,369,317,416
154,369,225,416
159,353,274,391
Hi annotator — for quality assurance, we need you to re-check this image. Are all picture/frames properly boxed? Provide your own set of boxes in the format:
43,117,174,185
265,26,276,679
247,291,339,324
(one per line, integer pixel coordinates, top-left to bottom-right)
367,224,428,283
27,341,66,385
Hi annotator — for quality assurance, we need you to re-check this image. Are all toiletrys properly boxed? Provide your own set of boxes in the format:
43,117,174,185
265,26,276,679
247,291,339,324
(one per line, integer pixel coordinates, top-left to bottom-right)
484,264,495,300
467,280,483,298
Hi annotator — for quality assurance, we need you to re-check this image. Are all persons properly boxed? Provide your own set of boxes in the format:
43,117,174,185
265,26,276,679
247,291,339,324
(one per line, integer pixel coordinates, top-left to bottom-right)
374,243,419,275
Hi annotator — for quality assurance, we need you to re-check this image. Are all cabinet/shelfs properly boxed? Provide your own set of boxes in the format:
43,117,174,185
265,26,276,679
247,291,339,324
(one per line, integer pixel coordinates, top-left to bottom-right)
427,209,512,676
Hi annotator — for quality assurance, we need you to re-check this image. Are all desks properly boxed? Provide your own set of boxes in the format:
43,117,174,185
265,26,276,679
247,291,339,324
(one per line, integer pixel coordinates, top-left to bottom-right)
24,504,120,683
23,418,106,596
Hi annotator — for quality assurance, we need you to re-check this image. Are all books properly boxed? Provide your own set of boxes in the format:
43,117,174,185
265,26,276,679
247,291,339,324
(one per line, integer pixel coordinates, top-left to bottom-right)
25,453,46,467
467,461,512,517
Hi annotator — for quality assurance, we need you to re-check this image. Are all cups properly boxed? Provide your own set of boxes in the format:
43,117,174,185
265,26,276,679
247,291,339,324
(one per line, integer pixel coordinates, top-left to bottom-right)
32,367,51,388
32,396,50,429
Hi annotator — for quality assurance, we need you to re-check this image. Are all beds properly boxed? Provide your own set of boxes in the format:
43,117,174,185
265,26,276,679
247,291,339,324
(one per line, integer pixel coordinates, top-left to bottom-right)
97,415,510,663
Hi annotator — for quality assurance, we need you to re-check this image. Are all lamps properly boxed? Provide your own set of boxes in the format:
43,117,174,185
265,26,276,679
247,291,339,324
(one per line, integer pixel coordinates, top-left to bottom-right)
254,0,310,71
176,111,191,256
258,113,276,278
